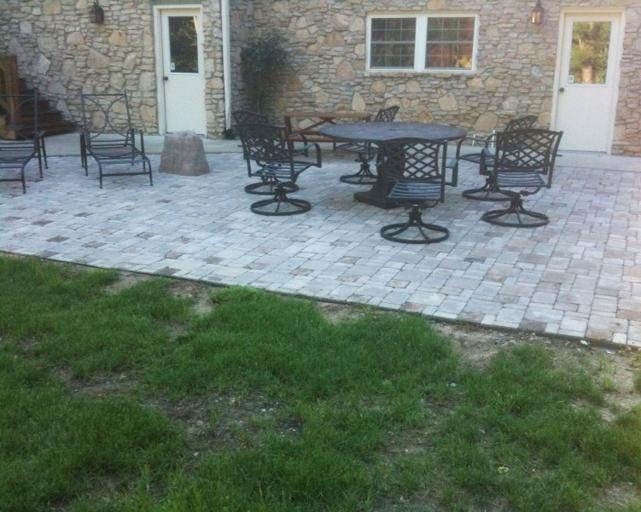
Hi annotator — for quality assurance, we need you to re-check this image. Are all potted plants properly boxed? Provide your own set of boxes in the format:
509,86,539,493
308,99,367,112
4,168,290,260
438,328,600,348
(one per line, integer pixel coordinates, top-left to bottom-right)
233,35,290,137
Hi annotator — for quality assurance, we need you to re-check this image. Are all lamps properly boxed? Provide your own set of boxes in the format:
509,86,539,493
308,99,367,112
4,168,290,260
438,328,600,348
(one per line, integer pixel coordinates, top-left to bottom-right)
531,0,544,24
88,0,104,23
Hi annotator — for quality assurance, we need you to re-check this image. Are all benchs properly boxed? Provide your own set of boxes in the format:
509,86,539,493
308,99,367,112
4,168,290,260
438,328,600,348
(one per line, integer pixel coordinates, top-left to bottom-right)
287,128,335,141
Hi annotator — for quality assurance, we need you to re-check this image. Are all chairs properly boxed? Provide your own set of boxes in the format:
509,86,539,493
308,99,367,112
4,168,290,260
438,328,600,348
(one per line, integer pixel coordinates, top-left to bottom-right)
236,124,322,216
77,88,153,189
340,105,400,185
375,138,458,243
482,129,564,227
231,110,310,195
0,88,48,194
456,115,537,201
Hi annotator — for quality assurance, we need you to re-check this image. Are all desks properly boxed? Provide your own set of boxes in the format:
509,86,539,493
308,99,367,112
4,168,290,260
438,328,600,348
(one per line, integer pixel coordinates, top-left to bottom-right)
282,112,371,151
320,122,467,210
